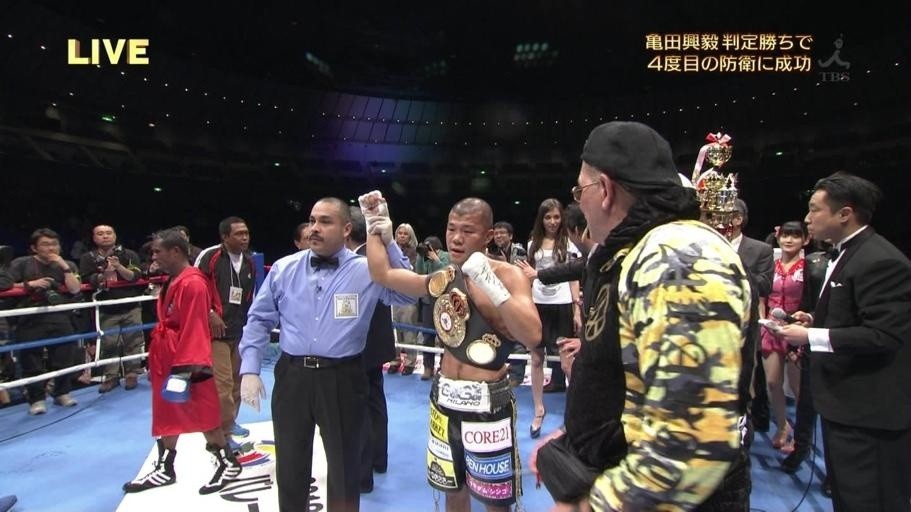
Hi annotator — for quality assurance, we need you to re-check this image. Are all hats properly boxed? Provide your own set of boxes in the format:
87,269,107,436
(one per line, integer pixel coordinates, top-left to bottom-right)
581,119,685,193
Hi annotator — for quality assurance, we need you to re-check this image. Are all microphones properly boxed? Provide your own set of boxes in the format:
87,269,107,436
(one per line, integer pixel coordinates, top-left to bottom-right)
772,307,798,324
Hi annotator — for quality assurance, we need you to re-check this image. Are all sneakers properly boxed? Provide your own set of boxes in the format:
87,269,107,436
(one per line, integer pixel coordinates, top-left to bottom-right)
53,395,80,408
97,377,140,392
778,447,810,472
542,381,567,395
226,423,250,452
25,401,48,416
419,366,436,382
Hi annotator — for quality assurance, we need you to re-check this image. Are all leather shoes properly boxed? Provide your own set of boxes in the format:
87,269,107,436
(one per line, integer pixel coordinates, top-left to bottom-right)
770,420,794,453
387,363,414,378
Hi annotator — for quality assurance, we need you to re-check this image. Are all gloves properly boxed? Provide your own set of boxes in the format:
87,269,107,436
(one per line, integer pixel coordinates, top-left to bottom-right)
160,373,191,403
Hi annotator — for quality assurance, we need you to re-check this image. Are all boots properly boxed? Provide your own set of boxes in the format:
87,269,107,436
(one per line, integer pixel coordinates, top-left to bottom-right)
122,438,176,492
198,444,245,496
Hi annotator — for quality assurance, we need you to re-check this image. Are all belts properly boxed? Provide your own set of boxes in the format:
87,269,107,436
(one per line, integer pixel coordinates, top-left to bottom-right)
281,349,360,370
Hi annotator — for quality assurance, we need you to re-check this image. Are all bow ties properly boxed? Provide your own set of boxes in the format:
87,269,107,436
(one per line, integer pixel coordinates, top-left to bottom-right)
308,254,341,268
825,246,841,260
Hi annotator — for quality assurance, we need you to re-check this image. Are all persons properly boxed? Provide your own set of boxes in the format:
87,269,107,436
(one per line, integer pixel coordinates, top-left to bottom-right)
2,122,911,512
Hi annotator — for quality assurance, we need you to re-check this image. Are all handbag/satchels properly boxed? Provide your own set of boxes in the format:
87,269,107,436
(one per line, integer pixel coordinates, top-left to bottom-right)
535,438,599,507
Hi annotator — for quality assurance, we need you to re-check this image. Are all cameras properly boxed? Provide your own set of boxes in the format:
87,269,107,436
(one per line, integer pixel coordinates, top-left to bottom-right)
95,256,107,272
488,244,505,255
416,242,432,256
36,280,61,305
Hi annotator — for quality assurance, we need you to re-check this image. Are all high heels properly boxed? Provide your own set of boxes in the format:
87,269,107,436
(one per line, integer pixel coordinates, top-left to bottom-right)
529,408,547,438
